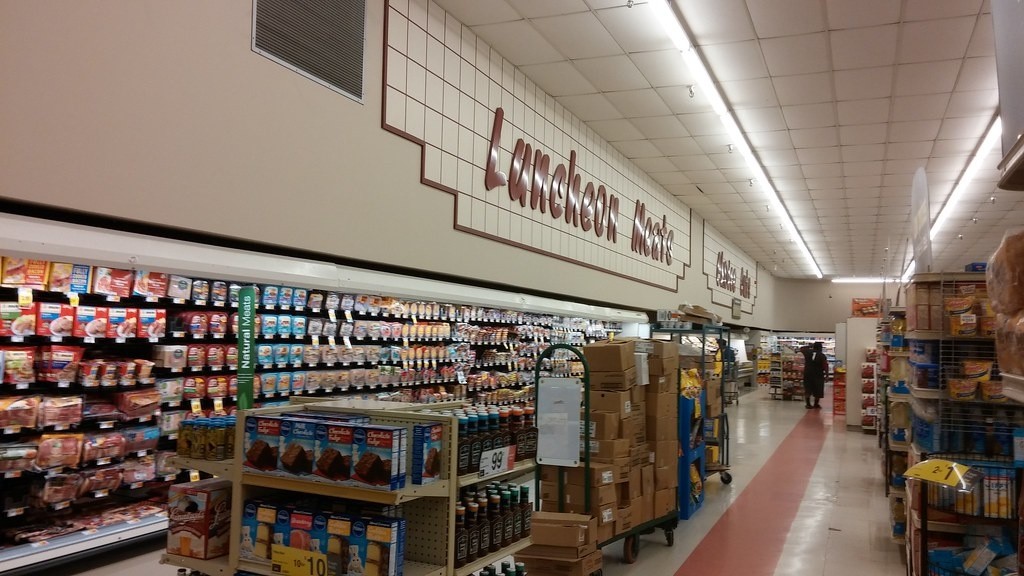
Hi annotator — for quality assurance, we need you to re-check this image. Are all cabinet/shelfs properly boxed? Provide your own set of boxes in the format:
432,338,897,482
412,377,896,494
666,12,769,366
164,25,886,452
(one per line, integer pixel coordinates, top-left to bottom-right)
860,133,1024,576
159,395,537,576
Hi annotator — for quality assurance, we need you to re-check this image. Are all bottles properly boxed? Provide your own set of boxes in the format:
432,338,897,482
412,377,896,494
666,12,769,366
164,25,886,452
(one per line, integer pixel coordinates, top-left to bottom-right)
467,561,528,576
455,482,533,568
418,405,538,475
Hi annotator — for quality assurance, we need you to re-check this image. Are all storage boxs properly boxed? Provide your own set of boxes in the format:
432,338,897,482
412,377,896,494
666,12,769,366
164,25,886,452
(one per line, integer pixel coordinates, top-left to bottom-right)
510,341,720,576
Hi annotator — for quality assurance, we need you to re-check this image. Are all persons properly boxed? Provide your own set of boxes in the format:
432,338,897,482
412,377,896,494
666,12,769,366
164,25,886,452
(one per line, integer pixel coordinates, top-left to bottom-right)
800,342,829,409
713,339,736,403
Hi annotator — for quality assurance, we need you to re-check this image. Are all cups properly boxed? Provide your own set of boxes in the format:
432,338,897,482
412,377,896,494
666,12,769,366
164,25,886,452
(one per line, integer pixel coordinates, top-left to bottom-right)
683,322,692,329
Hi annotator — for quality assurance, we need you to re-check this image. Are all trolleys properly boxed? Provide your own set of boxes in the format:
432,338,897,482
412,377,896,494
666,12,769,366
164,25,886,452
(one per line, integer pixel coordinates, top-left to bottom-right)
675,323,733,484
725,379,738,408
533,341,679,563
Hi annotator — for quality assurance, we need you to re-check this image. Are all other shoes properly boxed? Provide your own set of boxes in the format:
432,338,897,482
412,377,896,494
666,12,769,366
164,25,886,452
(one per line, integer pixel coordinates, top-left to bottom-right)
805,404,821,409
